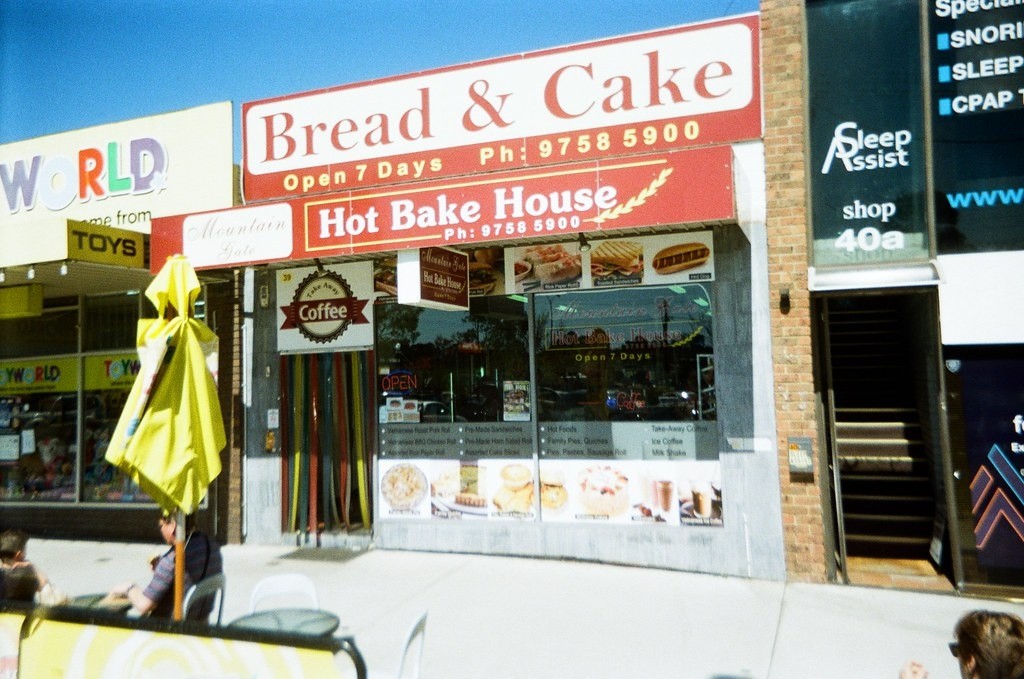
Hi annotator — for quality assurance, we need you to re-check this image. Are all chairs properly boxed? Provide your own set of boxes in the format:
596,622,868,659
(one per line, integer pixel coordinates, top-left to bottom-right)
172,573,225,627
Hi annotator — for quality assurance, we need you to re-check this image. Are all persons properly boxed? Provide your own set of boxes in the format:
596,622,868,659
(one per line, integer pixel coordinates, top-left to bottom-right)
114,502,222,626
0,529,60,606
897,611,1024,679
605,369,660,409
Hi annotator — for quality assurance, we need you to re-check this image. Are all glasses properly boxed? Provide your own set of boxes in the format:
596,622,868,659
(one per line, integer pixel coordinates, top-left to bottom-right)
949,643,961,657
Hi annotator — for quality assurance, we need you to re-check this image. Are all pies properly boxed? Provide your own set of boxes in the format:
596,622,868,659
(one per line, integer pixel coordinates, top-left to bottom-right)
524,246,584,283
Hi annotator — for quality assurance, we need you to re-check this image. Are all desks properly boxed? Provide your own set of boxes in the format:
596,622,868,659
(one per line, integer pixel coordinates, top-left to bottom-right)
227,609,367,679
51,593,133,616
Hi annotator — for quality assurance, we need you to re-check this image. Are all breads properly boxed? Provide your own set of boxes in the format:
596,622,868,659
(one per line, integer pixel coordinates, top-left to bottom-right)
590,240,643,266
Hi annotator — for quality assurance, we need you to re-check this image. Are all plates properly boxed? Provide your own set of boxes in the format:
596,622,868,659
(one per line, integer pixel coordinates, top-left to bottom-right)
513,259,532,282
380,463,429,511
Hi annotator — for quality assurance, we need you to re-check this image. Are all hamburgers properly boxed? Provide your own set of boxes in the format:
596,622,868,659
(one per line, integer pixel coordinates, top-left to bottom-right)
374,257,398,295
467,262,497,293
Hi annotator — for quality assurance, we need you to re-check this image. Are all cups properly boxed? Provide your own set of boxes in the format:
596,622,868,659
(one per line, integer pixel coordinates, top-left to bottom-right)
691,481,714,519
651,477,660,507
657,479,677,512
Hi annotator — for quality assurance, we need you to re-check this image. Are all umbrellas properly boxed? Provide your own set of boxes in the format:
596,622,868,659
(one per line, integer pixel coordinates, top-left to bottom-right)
104,252,226,624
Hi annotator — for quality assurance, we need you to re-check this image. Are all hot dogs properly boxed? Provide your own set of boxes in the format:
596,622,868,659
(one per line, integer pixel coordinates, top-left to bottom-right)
652,242,711,274
